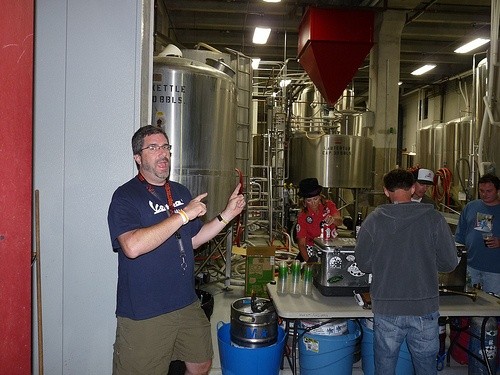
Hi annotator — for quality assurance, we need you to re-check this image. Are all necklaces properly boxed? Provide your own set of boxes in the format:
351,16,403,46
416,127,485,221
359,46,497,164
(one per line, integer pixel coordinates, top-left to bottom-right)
137,171,180,237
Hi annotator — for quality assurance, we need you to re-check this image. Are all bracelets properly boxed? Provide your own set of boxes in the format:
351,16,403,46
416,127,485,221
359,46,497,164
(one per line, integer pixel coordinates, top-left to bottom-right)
216,215,228,225
177,209,189,225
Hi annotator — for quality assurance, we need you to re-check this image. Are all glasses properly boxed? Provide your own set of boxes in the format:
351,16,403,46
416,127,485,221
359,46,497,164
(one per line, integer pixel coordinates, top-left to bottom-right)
139,144,171,153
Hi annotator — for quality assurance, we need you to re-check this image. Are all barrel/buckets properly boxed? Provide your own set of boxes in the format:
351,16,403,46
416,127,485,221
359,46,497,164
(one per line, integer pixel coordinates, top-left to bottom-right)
359,319,415,375
216,321,287,375
451,315,500,375
297,320,361,375
230,297,278,349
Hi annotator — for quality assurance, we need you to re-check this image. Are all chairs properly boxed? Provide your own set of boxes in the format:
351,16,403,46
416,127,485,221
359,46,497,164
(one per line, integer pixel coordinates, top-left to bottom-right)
409,22,490,76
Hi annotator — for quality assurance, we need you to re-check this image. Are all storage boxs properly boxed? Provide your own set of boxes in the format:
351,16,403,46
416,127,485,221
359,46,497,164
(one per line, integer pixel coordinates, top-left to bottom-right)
438,241,468,296
245,246,276,299
312,237,370,297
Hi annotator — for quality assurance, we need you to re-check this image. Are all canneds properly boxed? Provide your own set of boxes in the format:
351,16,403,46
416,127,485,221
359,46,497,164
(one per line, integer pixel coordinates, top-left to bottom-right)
323,224,332,240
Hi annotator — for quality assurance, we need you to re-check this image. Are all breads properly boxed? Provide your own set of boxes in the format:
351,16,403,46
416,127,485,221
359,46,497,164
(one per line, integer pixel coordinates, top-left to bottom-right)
362,292,370,303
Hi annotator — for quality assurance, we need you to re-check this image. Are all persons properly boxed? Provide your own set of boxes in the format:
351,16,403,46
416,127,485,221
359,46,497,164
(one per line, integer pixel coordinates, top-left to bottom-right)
455,175,500,375
295,177,343,264
410,168,434,204
107,125,246,375
354,169,459,375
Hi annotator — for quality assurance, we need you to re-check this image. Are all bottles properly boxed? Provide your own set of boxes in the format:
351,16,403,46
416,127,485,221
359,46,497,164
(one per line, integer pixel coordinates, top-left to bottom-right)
480,222,493,245
355,213,363,239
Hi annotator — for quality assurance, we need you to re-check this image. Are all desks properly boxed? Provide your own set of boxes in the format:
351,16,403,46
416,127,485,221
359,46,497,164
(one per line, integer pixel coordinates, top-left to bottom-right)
265,276,500,375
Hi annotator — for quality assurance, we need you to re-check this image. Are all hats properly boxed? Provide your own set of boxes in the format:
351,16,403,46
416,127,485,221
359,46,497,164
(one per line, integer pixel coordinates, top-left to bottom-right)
414,168,435,185
297,178,322,198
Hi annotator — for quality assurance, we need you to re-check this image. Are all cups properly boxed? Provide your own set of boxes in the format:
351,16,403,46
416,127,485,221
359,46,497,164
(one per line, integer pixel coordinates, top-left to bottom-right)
276,260,313,294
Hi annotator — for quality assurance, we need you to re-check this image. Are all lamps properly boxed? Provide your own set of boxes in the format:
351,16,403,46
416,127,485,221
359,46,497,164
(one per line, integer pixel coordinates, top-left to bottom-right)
249,13,291,88
